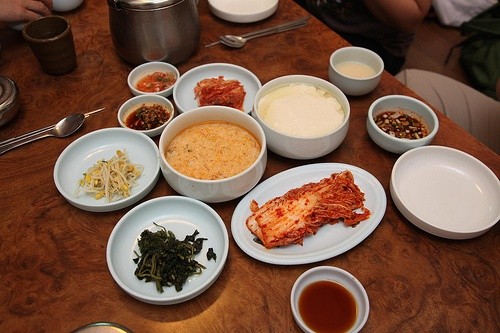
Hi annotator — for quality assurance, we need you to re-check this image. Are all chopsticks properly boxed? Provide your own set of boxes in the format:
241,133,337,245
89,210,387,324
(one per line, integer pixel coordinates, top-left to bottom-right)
204,16,312,47
0,107,105,148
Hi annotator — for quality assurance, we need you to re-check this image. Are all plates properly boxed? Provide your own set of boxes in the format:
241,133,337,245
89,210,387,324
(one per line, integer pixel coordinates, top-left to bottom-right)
208,0,279,23
290,266,369,333
172,63,261,115
106,196,229,305
231,163,387,265
390,146,500,240
53,127,160,213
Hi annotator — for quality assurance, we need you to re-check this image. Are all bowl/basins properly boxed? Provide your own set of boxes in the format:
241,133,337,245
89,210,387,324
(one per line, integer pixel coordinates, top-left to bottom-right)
127,61,180,97
252,74,351,160
158,105,268,203
366,95,439,154
117,95,174,137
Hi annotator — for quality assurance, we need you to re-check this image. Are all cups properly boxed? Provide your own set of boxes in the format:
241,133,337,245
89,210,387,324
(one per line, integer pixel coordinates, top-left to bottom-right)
328,46,384,95
22,15,77,76
51,0,83,12
106,0,200,67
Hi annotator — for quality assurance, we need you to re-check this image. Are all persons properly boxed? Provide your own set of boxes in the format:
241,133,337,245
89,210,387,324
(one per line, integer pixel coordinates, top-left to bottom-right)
393,67,500,159
287,0,432,76
0,0,53,27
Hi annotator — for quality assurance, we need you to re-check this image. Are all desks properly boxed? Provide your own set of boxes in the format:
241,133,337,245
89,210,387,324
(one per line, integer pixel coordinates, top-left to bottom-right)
1,1,500,333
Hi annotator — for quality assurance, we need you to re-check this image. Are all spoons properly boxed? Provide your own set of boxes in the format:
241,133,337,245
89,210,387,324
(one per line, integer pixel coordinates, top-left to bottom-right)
218,21,307,48
0,113,85,155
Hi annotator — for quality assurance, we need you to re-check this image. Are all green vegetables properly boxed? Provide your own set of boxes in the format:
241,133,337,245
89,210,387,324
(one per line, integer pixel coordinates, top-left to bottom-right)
132,222,216,293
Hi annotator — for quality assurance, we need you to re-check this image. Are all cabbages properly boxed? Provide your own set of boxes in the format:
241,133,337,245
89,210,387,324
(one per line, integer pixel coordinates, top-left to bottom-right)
246,170,370,250
